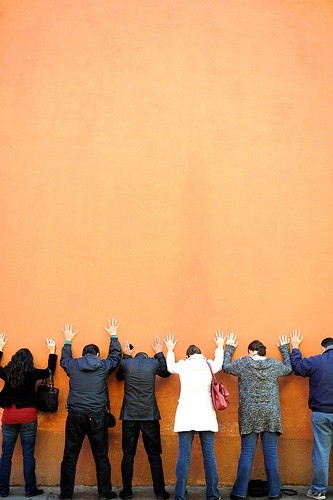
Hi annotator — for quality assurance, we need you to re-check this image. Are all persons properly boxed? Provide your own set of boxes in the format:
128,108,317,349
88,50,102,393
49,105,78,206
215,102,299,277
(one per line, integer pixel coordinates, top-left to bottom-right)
290,329,333,500
222,333,293,500
0,333,58,498
60,318,224,500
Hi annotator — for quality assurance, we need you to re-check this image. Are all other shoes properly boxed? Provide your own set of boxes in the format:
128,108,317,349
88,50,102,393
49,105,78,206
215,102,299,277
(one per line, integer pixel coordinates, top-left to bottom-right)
217,496,223,500
230,492,248,500
269,493,282,500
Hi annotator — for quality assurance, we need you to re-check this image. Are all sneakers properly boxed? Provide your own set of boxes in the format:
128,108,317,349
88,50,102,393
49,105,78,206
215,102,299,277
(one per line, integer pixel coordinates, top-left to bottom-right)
25,486,44,497
157,492,170,500
98,491,117,500
59,493,72,500
0,490,8,496
305,488,326,500
119,491,132,500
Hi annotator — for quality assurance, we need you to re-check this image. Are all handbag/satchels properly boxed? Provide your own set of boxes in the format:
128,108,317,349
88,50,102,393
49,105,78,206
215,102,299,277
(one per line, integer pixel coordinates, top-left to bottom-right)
35,367,59,413
207,362,231,411
105,412,116,427
247,480,297,497
